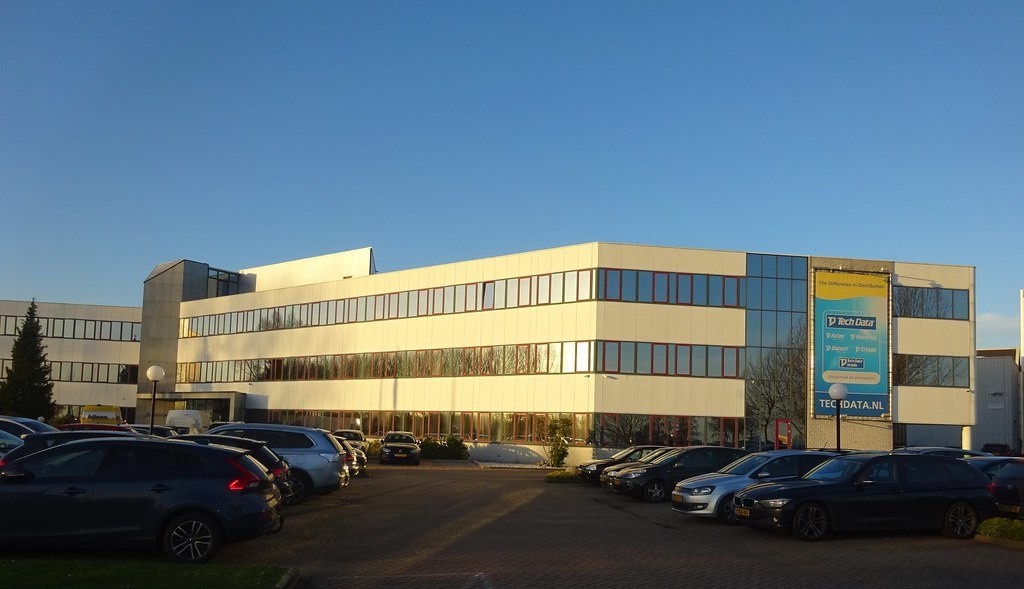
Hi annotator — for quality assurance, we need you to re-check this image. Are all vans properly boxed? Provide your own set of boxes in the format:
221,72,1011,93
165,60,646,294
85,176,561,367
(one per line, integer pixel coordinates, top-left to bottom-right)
166,409,202,436
80,404,123,425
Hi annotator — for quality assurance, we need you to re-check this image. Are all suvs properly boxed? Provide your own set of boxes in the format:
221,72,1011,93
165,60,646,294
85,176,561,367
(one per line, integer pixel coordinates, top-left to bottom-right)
0,414,366,564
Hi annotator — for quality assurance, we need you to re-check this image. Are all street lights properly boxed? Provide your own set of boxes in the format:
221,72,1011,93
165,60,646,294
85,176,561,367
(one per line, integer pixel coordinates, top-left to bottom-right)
829,383,848,451
145,366,164,437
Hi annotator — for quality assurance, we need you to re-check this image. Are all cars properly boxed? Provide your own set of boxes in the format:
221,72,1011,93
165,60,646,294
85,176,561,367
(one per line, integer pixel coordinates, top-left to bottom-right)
670,449,849,527
732,452,1000,540
599,447,676,488
576,444,667,480
614,445,748,502
960,458,1024,523
380,431,420,465
